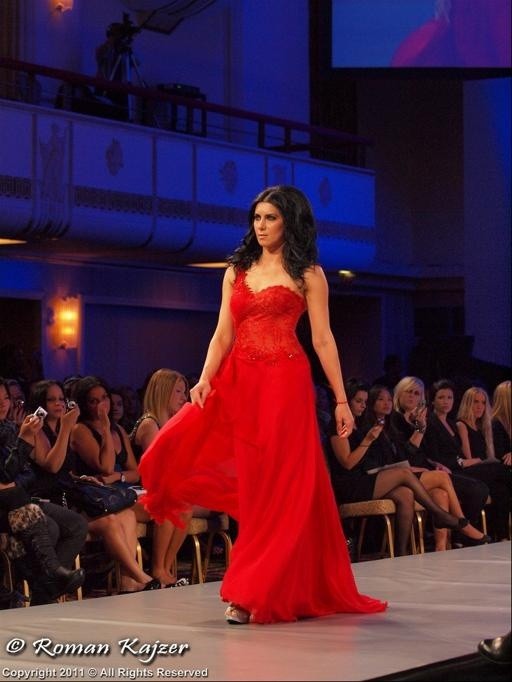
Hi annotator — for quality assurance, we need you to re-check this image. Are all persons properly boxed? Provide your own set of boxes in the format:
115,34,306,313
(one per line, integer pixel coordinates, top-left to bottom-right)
0,368,511,611
188,186,357,625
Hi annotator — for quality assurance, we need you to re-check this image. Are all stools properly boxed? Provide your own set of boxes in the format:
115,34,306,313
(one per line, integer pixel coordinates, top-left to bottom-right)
0,498,511,607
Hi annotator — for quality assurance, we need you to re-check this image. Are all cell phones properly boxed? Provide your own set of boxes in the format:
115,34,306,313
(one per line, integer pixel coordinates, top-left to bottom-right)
65,398,77,414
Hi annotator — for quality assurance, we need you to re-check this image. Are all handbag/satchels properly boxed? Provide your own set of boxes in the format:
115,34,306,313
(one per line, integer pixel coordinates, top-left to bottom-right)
69,476,138,517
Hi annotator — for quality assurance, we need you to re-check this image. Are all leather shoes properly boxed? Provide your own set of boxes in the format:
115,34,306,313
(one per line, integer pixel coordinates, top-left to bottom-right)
83,558,115,592
457,529,491,544
476,631,511,665
434,517,467,530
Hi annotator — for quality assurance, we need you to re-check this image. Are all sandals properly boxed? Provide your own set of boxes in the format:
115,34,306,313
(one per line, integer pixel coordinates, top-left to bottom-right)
224,605,250,623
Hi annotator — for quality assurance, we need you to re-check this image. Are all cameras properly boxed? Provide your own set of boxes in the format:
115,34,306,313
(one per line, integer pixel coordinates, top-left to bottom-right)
29,406,48,421
416,399,426,410
13,394,26,408
377,417,385,424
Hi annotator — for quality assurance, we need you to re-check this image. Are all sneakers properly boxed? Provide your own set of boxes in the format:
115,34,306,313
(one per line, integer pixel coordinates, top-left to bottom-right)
118,576,191,594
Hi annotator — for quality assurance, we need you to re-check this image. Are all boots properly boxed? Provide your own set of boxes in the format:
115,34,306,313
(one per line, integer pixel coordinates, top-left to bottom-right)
20,525,85,603
